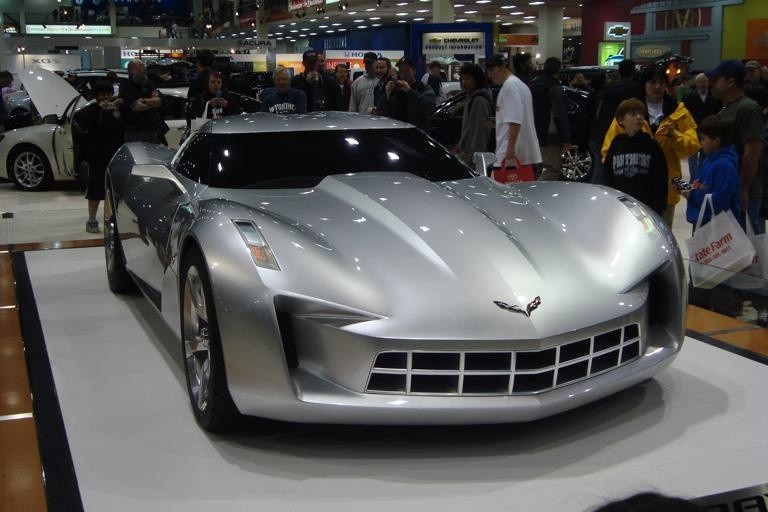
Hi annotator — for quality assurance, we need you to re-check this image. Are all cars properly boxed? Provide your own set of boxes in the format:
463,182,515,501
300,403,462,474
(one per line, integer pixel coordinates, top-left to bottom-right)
421,52,695,178
1,61,269,191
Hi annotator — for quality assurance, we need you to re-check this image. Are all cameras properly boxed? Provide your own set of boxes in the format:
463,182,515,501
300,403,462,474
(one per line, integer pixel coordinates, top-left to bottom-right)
391,76,401,88
106,102,117,110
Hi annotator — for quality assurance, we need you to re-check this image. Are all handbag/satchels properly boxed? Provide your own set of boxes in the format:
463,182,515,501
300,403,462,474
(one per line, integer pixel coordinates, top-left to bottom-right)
494,165,535,183
684,208,757,290
190,117,212,133
721,233,768,290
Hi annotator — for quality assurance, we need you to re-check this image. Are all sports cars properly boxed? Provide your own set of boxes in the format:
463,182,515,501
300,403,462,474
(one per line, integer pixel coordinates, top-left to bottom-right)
103,108,692,441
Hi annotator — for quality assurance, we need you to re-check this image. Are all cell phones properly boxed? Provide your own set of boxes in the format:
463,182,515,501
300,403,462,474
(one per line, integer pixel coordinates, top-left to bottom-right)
215,90,224,98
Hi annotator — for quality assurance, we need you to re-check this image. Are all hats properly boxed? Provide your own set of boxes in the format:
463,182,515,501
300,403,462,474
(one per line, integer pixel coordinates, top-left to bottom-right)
486,54,506,67
744,60,761,70
395,56,416,67
364,52,377,64
303,50,319,63
704,59,747,83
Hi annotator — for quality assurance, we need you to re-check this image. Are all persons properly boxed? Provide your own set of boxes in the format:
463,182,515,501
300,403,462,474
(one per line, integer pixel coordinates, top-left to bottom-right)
67,48,766,235
670,114,740,319
49,0,235,38
0,70,13,132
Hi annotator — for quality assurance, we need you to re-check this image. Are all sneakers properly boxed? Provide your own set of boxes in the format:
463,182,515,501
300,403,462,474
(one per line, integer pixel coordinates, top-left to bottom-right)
86,220,100,233
